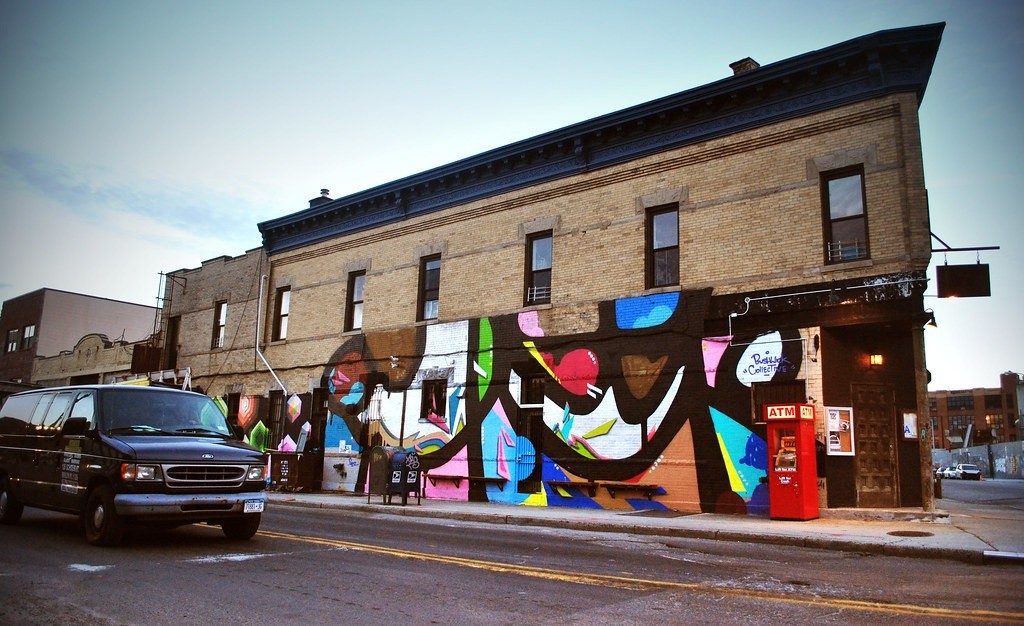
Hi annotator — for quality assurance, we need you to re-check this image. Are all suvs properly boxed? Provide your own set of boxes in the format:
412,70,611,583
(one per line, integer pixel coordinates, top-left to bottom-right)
0,384,273,548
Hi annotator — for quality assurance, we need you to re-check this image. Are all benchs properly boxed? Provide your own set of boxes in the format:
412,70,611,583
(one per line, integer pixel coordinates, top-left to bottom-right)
547,481,599,497
601,481,657,499
471,476,507,492
422,474,463,488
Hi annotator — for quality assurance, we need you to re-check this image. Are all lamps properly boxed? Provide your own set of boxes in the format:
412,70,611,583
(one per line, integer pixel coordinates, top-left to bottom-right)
871,348,883,370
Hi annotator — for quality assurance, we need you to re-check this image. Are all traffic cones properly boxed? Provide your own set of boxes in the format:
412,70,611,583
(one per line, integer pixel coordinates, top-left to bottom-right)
980,475,983,481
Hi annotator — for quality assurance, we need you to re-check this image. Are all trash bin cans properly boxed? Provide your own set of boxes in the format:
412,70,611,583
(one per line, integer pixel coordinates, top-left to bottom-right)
368,446,421,506
270,451,316,486
933,474,942,498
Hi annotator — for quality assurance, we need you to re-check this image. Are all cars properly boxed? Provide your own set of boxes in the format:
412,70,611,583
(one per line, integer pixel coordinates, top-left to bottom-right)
933,469,937,479
936,467,947,479
943,466,957,479
955,463,982,480
